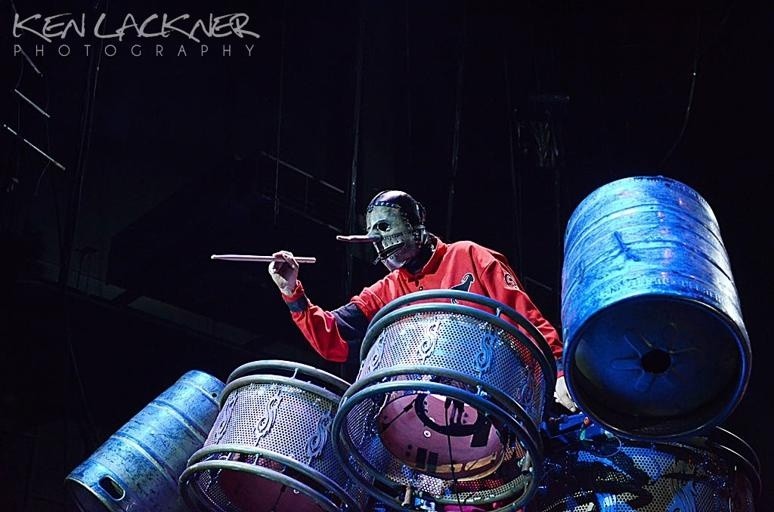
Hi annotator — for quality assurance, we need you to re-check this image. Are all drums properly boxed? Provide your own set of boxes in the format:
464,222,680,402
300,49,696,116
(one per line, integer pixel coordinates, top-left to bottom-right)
540,415,762,512
561,176,752,441
178,360,370,512
331,288,557,512
65,370,225,512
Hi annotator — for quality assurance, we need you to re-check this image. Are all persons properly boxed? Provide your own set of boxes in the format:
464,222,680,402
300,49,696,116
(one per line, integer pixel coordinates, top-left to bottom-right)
268,186,581,412
